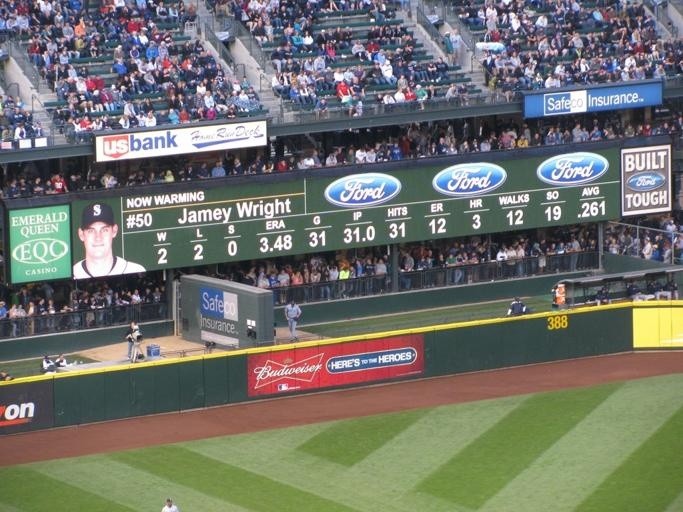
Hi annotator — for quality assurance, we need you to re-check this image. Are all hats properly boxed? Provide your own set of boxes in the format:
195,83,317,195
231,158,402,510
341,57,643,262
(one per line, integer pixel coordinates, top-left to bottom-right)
80,202,114,226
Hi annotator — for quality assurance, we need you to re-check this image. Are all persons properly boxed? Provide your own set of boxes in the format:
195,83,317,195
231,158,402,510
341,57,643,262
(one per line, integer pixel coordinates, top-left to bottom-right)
162,499,178,512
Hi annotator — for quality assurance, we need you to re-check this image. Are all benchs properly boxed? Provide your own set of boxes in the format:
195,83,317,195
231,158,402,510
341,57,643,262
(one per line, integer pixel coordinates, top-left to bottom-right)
551,290,682,308
0,1,676,148
